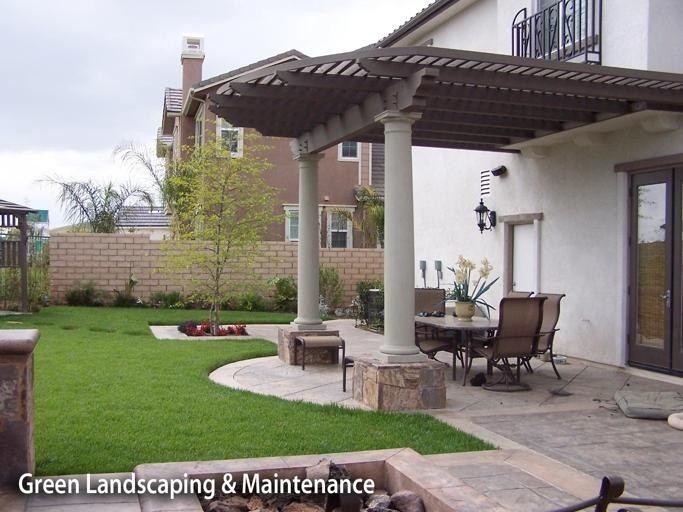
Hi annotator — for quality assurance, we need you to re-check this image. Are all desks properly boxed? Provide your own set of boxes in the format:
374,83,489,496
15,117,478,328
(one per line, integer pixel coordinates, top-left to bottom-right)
414,315,498,380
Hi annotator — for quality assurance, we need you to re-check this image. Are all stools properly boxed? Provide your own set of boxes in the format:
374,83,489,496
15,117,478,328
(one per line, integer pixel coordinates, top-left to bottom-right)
342,355,354,392
294,335,345,371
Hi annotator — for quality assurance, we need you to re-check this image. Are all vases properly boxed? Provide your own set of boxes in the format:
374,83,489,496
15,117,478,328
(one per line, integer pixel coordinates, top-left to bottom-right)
454,302,474,322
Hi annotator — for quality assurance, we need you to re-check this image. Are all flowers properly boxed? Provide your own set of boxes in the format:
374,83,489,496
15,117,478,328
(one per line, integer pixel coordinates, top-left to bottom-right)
433,255,499,319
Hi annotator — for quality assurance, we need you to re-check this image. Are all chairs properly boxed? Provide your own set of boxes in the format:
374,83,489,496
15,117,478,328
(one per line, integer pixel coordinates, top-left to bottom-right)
520,293,565,379
482,291,534,392
461,297,547,386
414,289,451,369
